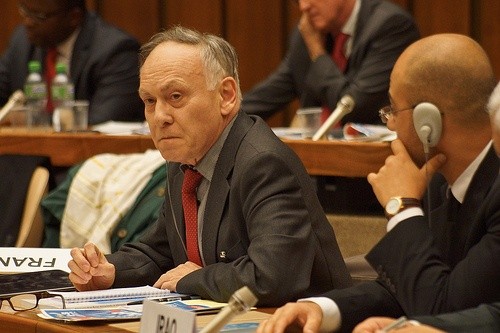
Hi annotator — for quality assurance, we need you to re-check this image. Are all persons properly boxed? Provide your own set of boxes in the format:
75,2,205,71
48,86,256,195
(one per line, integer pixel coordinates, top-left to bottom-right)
256,33,500,333
68,24,355,309
0,0,149,247
237,0,422,217
354,83,500,333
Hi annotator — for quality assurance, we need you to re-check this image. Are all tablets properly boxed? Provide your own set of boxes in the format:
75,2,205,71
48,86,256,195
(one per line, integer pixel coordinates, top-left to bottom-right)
0,269,75,299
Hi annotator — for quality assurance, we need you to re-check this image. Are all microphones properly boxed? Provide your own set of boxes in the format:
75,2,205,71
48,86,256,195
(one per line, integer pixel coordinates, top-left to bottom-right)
200,286,259,333
312,93,358,142
1,89,27,128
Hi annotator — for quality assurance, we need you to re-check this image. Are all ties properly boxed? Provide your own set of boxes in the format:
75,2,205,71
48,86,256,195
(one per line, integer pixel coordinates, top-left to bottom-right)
322,32,347,127
41,49,60,112
181,168,203,266
446,191,459,243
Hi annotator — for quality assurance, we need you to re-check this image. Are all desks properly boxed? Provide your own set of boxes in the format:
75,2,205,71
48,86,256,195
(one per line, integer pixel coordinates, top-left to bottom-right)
0,287,303,333
1,126,394,218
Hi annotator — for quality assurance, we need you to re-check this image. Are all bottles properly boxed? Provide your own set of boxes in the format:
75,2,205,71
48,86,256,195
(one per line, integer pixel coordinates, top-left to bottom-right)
23,61,49,131
50,64,75,133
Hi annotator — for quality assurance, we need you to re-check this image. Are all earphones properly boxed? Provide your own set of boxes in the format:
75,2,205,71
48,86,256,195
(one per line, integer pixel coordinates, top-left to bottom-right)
412,102,443,147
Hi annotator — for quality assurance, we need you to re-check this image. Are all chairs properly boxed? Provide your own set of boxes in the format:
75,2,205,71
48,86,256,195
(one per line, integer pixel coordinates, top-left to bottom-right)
14,165,48,248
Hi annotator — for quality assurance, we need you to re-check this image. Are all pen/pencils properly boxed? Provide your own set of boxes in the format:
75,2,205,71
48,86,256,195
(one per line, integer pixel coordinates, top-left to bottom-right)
125,293,192,308
383,316,407,333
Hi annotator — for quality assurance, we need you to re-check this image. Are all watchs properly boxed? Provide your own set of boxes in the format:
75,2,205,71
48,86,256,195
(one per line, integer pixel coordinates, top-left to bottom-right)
384,196,423,221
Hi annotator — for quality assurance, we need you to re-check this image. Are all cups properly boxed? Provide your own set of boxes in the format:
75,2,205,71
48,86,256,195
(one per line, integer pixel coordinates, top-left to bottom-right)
8,96,30,128
296,109,323,140
65,101,89,129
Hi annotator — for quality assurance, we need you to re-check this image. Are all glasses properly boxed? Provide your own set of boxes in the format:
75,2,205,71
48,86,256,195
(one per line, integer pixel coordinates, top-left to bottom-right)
379,103,443,124
16,4,62,22
0,290,67,314
